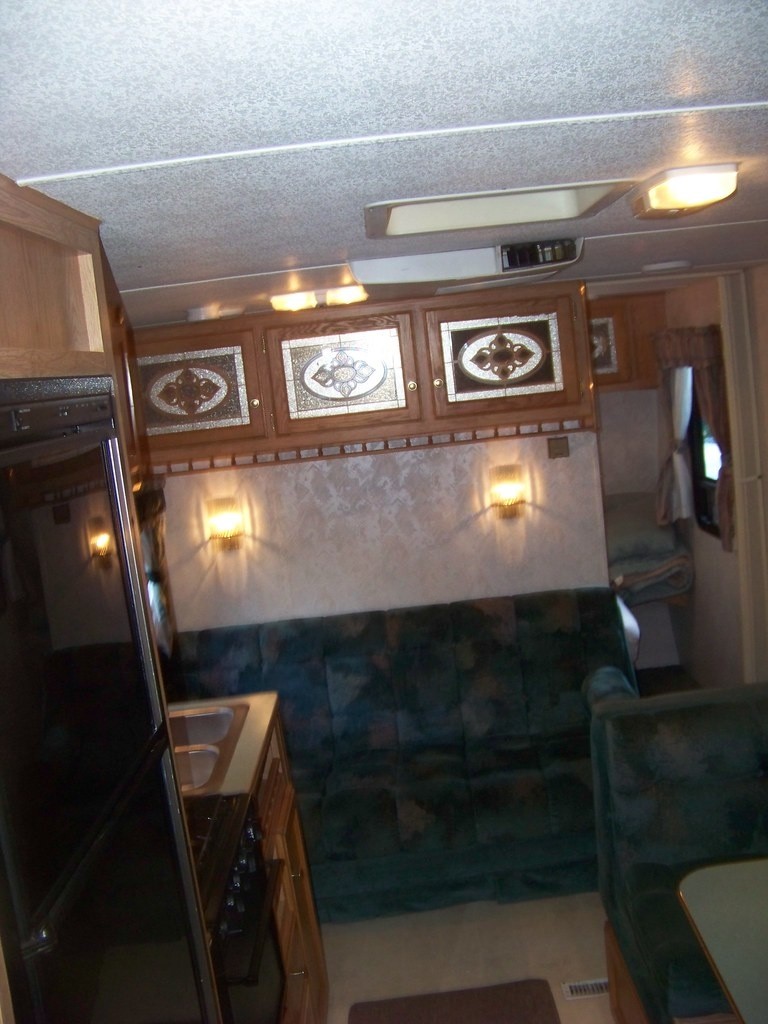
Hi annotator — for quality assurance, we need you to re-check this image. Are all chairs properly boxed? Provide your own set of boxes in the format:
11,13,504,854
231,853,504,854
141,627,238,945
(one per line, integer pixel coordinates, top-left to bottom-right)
587,679,768,1024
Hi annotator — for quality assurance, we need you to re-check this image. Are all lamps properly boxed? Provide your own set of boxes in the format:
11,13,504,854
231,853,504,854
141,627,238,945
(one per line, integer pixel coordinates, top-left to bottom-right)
490,464,526,519
628,160,741,220
206,496,243,552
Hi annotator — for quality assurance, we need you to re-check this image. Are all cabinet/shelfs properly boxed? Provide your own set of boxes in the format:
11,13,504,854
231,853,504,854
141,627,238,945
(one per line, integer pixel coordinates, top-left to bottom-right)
126,281,665,466
209,717,329,1024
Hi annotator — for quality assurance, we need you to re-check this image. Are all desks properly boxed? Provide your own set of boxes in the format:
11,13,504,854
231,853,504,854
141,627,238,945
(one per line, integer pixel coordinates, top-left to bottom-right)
676,858,768,1022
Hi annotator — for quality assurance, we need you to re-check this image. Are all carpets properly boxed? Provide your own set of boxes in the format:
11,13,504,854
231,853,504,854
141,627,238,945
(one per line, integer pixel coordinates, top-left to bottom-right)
349,978,561,1024
